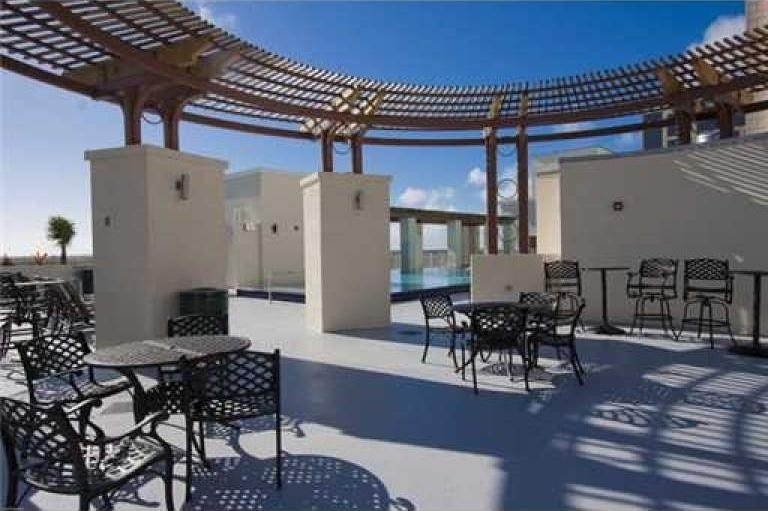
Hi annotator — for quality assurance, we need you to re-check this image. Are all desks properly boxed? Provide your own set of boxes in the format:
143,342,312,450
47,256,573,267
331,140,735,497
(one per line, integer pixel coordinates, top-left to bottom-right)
85,337,253,440
579,265,631,334
721,270,767,356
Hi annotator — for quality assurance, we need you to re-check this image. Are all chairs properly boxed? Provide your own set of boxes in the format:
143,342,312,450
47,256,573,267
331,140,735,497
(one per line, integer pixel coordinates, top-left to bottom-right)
165,313,232,338
16,331,134,433
2,272,96,367
177,348,281,485
627,257,680,337
0,395,191,504
418,260,587,395
674,258,736,348
